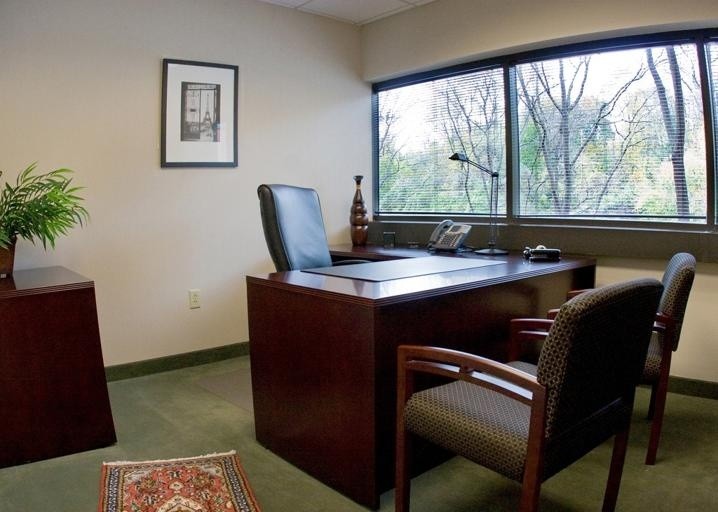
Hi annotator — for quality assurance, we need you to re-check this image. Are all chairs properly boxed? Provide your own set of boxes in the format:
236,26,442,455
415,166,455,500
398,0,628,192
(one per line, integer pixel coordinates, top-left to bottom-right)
395,277,665,512
256,184,374,272
546,252,697,466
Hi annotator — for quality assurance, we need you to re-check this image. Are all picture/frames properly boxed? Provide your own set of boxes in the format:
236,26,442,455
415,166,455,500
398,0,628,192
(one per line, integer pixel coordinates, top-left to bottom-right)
160,58,240,169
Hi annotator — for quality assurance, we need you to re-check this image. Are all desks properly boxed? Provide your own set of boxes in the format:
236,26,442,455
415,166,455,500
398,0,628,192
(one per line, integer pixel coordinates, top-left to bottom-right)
0,266,119,470
246,242,598,512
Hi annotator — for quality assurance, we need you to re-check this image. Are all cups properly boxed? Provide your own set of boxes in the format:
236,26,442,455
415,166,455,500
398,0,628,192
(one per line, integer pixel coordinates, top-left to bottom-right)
382,231,397,249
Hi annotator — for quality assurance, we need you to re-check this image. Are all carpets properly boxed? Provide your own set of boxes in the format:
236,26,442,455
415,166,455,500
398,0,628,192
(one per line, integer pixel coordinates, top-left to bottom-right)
97,449,262,511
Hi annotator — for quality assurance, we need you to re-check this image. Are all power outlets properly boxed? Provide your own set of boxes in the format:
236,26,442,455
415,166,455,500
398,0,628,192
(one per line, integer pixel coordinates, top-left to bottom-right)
189,288,202,309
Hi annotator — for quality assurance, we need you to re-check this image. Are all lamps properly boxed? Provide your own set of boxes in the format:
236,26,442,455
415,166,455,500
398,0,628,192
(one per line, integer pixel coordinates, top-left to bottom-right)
449,152,511,256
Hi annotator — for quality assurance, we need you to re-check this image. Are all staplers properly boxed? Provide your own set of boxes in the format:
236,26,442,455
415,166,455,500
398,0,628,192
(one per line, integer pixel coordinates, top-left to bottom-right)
529,248,561,262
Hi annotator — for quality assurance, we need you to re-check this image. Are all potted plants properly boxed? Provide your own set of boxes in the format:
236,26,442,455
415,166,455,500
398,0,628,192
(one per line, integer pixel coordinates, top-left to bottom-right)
0,159,92,278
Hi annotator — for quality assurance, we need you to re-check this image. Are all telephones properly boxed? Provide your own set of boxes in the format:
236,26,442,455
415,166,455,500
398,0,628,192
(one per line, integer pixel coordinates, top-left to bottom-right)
428,220,472,250
